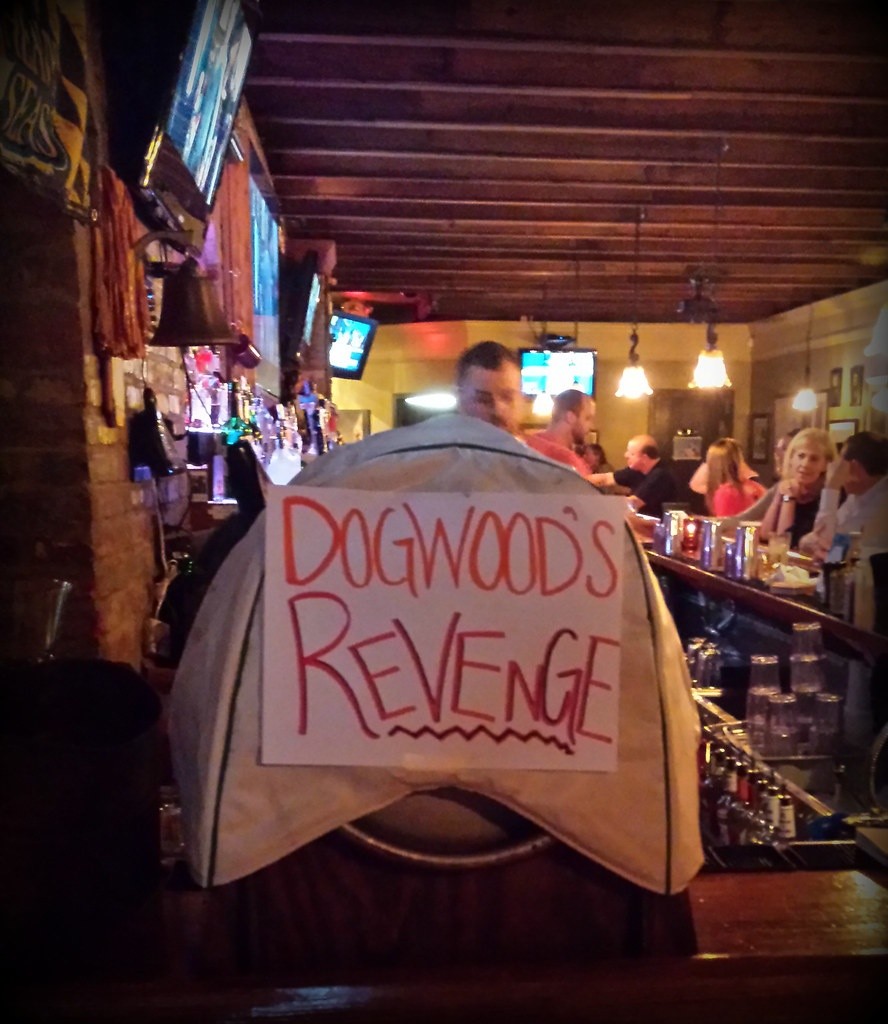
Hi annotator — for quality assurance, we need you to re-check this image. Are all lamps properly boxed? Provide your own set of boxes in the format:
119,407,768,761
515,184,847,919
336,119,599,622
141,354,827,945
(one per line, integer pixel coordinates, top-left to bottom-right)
676,273,719,326
612,208,655,400
687,138,732,393
234,333,262,368
790,309,820,412
530,253,587,417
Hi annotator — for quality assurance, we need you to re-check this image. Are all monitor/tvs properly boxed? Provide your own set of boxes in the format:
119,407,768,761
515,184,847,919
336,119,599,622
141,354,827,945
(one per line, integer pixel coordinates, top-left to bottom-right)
519,348,598,400
282,253,321,368
328,312,379,380
131,0,255,254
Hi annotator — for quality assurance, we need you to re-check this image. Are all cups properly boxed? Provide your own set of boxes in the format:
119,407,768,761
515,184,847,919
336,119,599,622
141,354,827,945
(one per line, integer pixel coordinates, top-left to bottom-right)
652,510,887,824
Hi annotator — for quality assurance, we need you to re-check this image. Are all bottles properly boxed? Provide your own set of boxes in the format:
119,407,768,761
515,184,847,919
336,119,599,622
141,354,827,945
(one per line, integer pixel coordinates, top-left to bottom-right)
693,736,797,876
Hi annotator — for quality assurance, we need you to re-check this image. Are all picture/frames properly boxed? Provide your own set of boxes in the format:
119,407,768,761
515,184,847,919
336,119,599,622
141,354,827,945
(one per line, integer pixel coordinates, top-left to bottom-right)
750,413,768,464
827,418,858,443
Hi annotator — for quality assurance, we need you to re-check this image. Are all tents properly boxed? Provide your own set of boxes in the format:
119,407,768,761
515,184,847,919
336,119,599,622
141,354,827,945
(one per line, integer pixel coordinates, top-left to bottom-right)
169,410,707,898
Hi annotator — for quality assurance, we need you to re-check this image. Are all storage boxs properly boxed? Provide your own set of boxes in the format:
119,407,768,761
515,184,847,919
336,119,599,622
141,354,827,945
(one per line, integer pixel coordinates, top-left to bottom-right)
672,436,702,459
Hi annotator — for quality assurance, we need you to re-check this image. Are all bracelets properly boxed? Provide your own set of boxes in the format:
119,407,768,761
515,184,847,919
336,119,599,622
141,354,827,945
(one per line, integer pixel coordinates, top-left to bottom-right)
783,496,797,502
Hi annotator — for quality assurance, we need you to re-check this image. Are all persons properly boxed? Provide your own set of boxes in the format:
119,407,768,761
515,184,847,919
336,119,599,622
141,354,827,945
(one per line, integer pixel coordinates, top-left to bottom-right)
574,427,888,593
533,389,595,475
453,341,590,479
